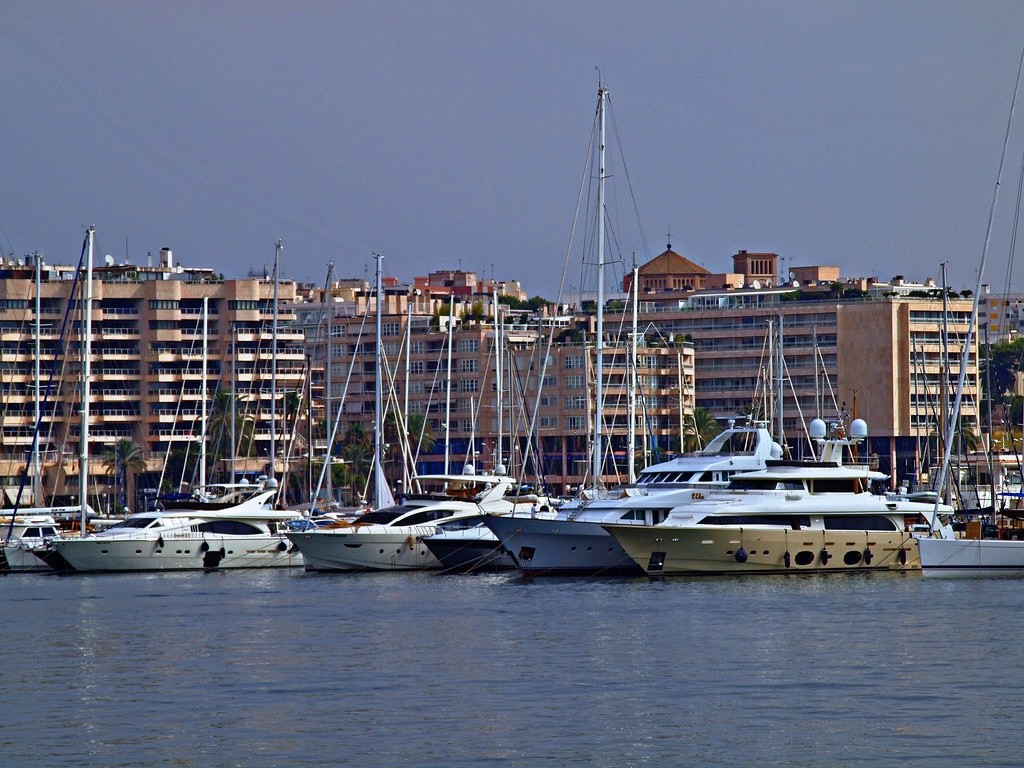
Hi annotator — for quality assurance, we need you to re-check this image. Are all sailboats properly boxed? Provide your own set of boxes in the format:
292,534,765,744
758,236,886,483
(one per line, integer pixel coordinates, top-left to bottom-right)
0,52,1024,574
